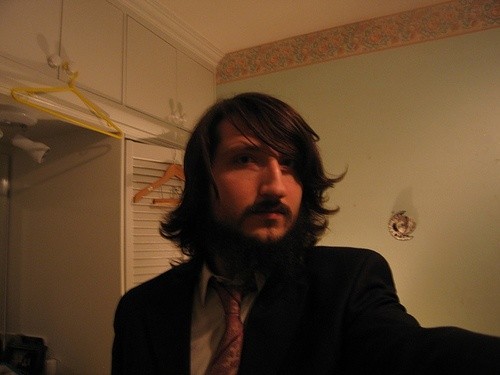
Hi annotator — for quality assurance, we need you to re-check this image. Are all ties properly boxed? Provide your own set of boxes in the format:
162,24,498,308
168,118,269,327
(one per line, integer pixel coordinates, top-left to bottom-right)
206,277,258,375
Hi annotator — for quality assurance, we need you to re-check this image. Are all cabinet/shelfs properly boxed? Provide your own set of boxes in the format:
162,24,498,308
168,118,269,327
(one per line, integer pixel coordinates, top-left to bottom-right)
123,11,218,131
0,0,123,103
10,130,194,375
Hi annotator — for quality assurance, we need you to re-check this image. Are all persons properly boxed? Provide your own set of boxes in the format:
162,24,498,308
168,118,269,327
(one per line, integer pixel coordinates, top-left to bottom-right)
113,92,500,375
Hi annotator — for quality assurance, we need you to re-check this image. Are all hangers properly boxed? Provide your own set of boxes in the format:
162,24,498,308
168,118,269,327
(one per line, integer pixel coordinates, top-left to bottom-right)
10,68,125,140
131,147,188,205
